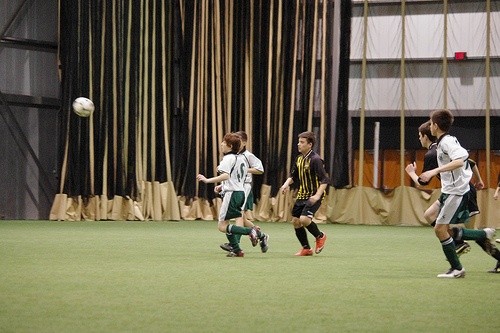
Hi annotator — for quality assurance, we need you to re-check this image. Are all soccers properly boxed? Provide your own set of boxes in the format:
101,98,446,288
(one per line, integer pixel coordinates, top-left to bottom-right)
72,97,94,117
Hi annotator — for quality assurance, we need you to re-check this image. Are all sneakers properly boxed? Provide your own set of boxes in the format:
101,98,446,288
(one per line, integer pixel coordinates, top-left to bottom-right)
250,226,258,247
294,247,313,256
226,250,244,257
437,267,465,279
446,242,471,261
259,233,269,253
220,243,233,251
482,227,496,256
493,261,500,273
315,232,327,254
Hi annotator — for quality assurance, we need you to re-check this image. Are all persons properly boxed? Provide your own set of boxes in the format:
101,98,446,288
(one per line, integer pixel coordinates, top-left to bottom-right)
494,173,500,243
214,132,269,253
405,122,500,273
282,132,329,256
419,110,496,277
196,133,260,256
